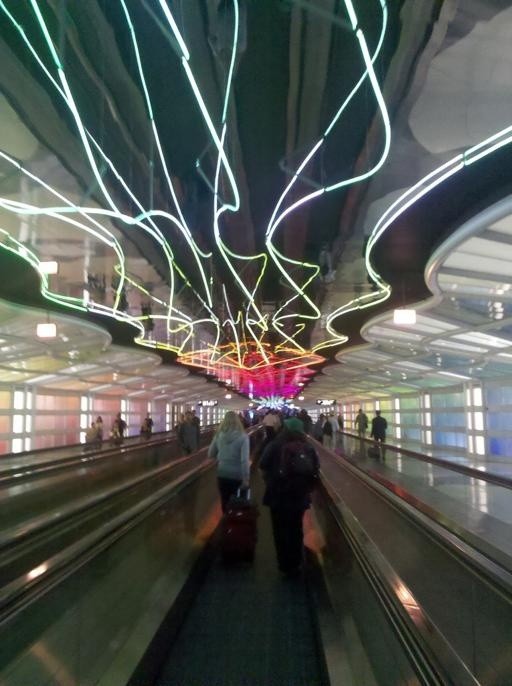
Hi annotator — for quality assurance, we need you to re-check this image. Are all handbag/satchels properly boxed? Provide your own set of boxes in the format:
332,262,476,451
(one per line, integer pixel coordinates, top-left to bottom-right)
323,417,333,434
221,493,255,511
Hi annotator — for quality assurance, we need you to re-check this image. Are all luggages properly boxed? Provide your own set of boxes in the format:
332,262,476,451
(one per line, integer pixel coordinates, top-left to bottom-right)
367,445,381,460
221,486,258,564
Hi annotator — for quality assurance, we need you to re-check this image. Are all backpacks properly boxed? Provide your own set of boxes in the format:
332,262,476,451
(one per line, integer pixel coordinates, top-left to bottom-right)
279,439,320,487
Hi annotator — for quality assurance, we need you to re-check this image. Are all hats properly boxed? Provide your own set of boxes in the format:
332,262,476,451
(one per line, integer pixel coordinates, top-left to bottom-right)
283,417,305,435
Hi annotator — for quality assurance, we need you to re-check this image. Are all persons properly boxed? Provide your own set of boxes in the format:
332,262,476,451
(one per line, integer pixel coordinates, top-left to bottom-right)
174,410,201,457
87,272,107,306
207,407,346,576
86,413,152,450
354,407,387,462
140,290,155,332
111,276,130,310
